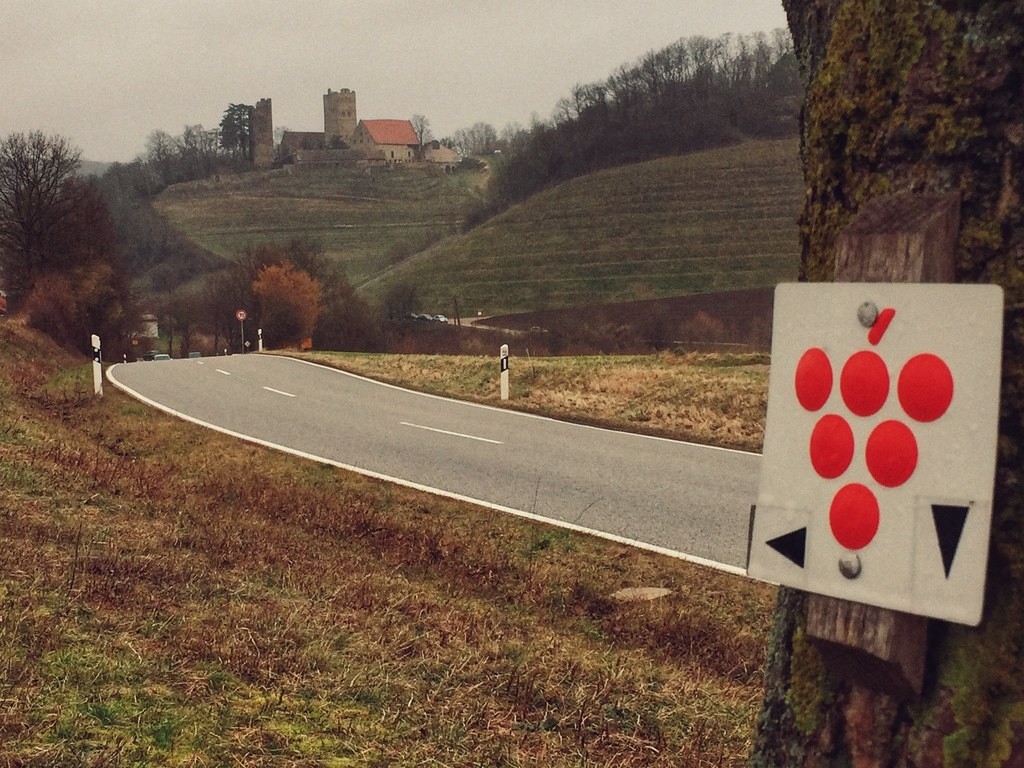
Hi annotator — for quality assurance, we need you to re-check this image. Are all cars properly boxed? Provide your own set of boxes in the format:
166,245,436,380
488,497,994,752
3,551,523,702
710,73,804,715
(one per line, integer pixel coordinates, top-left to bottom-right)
152,354,174,361
